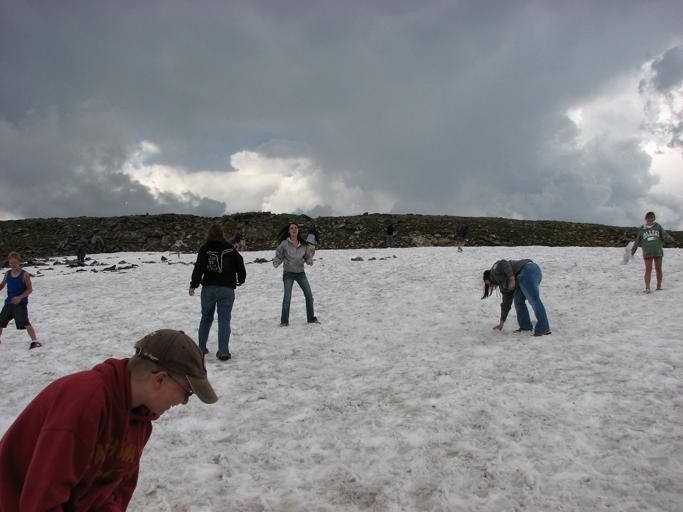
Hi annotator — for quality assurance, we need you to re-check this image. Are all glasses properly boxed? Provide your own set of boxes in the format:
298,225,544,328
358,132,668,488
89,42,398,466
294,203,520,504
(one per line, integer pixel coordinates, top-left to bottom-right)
153,370,193,400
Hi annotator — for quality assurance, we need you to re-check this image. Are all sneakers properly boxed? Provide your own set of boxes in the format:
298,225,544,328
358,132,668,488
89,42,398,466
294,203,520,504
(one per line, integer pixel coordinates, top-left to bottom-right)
30,341,41,349
219,354,229,361
512,328,551,336
280,317,321,328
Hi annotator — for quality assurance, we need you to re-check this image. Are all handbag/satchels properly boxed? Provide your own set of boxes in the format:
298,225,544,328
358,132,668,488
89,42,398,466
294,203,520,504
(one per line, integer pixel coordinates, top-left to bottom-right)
306,234,317,246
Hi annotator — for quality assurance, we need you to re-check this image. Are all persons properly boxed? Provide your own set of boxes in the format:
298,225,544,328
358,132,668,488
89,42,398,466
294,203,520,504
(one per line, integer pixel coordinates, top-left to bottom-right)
453,223,466,253
0,329,221,512
240,227,246,251
187,222,246,362
233,230,244,251
271,221,321,328
480,257,553,337
305,223,321,257
630,211,678,294
0,251,42,350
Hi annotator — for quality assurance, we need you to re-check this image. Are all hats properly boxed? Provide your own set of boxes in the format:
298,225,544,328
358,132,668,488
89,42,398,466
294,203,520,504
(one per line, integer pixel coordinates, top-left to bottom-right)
136,329,218,403
